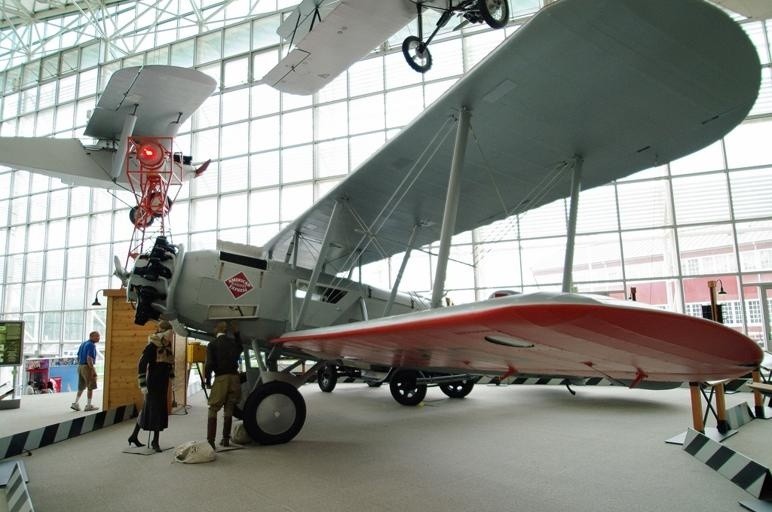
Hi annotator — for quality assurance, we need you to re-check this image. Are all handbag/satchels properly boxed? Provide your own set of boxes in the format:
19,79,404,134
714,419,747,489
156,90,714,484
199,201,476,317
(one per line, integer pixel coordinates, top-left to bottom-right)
174,441,216,464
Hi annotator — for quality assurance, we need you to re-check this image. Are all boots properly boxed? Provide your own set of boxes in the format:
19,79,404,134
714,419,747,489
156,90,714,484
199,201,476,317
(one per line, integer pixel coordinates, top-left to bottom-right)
207,417,216,449
220,416,232,446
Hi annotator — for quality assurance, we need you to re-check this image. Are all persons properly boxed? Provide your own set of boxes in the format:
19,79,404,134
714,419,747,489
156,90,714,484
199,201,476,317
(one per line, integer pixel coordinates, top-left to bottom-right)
71,332,100,411
205,320,244,450
47,382,54,393
128,320,176,452
26,381,34,395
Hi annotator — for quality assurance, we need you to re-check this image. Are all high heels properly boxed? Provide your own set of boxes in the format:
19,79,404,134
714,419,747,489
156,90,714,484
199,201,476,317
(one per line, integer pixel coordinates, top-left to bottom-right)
151,441,161,452
128,436,145,447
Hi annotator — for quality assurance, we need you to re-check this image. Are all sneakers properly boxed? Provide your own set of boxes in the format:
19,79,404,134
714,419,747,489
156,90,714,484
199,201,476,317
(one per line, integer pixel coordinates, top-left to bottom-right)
85,405,98,411
71,403,80,410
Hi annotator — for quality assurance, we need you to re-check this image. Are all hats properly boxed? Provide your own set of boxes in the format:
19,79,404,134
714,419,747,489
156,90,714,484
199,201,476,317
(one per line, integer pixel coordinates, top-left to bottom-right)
158,320,172,332
215,321,227,333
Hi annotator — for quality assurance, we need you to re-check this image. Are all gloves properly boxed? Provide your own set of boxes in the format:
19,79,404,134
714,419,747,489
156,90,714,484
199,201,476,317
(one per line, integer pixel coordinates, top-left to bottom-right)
140,387,148,394
